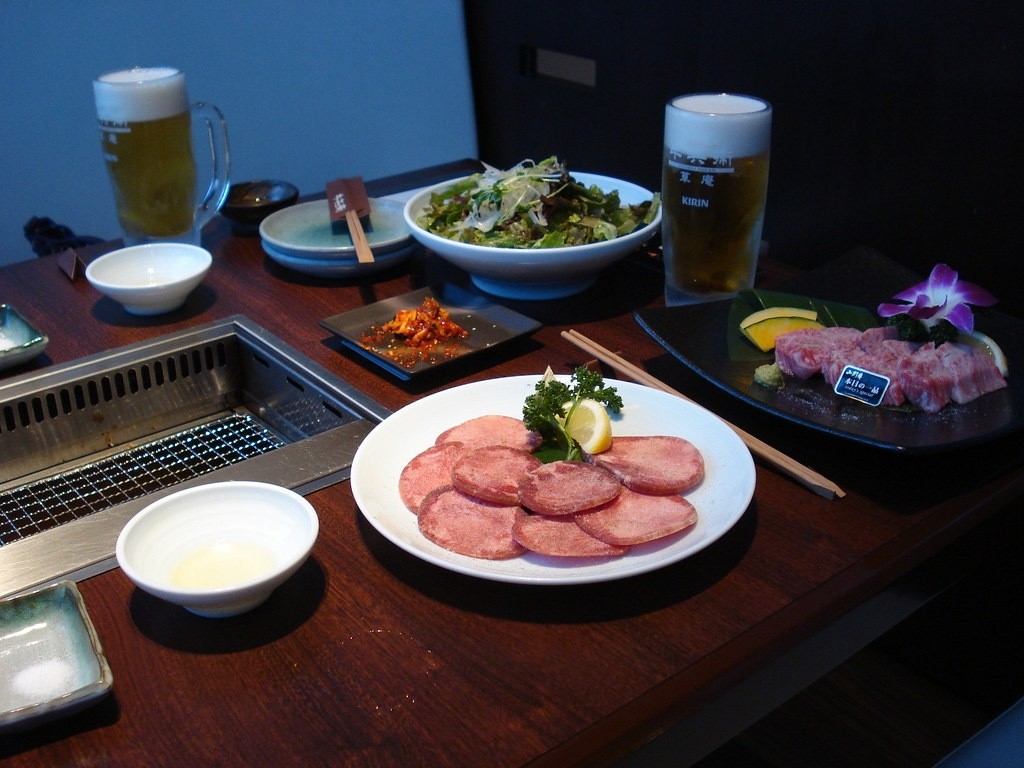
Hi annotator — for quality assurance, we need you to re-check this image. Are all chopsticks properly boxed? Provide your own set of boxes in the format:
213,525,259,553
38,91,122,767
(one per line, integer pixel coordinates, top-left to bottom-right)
346,210,376,263
561,329,846,500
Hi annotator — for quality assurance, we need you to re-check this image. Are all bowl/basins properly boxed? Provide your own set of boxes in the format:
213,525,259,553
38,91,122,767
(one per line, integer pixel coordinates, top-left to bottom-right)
220,180,299,221
115,481,319,618
84,242,212,314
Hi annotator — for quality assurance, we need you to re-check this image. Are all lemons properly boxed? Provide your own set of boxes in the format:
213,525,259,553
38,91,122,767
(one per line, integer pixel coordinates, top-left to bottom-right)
554,397,612,454
952,329,1007,378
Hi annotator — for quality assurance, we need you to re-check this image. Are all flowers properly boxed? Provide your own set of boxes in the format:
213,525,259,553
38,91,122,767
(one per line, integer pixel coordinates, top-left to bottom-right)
874,263,999,336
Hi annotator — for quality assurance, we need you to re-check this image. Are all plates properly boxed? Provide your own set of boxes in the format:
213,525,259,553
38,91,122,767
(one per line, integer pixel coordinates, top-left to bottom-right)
350,374,756,585
260,197,423,278
0,304,49,368
0,580,114,727
404,170,663,300
316,281,543,381
635,288,1024,450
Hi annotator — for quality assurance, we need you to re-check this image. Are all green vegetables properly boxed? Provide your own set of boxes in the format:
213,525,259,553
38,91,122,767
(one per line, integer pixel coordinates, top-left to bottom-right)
521,362,623,462
417,156,660,250
879,314,959,350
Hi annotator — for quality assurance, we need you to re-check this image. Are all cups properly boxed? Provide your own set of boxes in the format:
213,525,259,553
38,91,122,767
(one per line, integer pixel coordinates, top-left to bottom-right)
92,66,232,249
661,92,774,306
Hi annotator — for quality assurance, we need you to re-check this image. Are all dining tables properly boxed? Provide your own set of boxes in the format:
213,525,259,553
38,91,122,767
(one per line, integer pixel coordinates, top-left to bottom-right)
0,157,1024,767
